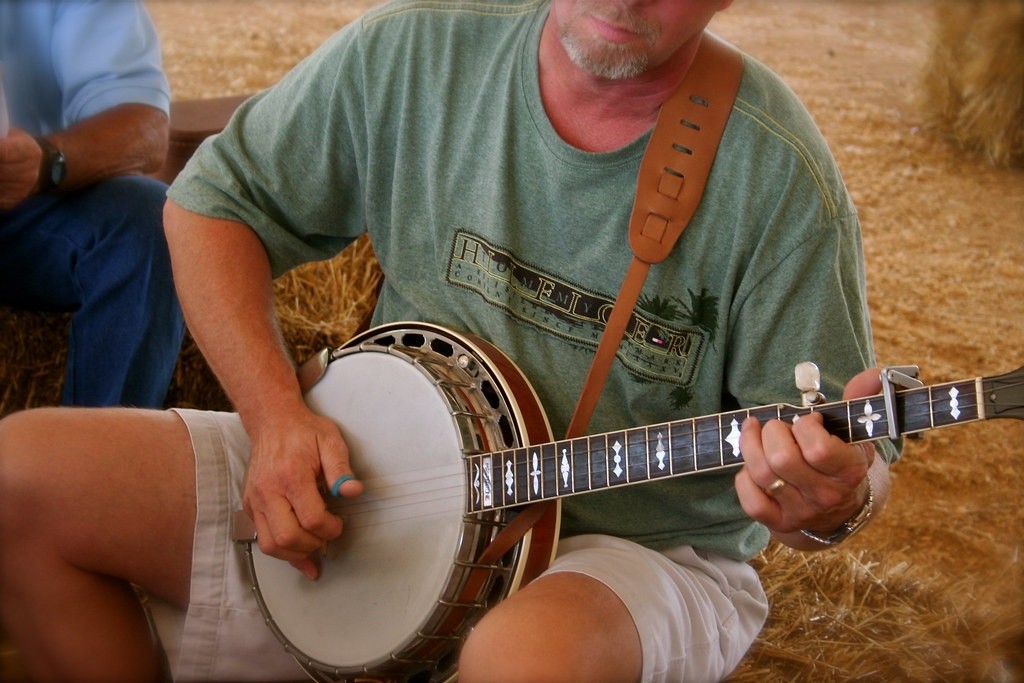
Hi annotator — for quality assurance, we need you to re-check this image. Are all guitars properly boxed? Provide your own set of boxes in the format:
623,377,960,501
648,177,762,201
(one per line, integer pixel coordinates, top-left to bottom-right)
230,319,1024,683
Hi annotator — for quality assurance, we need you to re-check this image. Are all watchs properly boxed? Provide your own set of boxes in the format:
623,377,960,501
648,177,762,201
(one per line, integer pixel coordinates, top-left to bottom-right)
32,137,66,198
800,474,873,546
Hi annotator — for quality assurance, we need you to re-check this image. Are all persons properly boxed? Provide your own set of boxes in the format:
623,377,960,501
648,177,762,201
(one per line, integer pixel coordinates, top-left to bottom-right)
0,0,903,683
0,0,185,410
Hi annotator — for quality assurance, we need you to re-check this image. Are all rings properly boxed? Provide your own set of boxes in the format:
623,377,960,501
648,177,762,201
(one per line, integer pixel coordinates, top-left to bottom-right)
765,479,786,499
332,474,357,499
